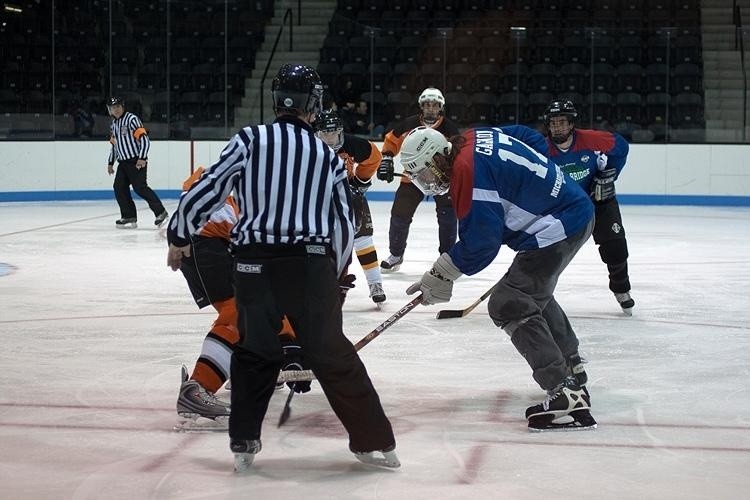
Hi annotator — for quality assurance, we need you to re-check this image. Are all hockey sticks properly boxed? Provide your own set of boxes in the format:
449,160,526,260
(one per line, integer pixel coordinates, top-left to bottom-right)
436,283,498,319
279,293,423,381
277,381,296,427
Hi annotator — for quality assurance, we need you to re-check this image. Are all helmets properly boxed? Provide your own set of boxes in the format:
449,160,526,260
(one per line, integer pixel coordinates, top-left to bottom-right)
349,183,364,236
106,96,125,116
311,108,344,154
400,126,452,196
418,87,445,124
544,98,579,144
272,63,323,125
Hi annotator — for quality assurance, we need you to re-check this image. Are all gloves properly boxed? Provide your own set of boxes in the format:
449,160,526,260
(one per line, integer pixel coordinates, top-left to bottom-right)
377,153,394,183
591,169,616,202
279,334,311,392
350,176,372,195
406,252,463,306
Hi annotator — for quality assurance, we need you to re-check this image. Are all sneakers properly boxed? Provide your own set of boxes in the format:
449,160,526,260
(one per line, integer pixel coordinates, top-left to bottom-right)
155,210,167,225
614,291,634,308
349,442,396,455
368,283,386,303
230,439,261,453
569,353,588,385
381,254,403,269
526,384,591,420
116,218,137,224
177,365,231,418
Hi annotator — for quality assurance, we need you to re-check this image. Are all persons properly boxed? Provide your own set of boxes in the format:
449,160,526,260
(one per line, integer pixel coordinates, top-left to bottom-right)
65,92,95,139
166,62,399,457
541,96,635,316
305,79,381,139
166,162,311,418
105,95,169,226
310,108,387,309
376,86,460,270
160,101,194,140
399,121,597,431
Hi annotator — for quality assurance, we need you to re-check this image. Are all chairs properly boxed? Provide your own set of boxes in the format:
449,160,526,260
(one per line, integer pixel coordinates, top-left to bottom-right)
0,1,276,131
